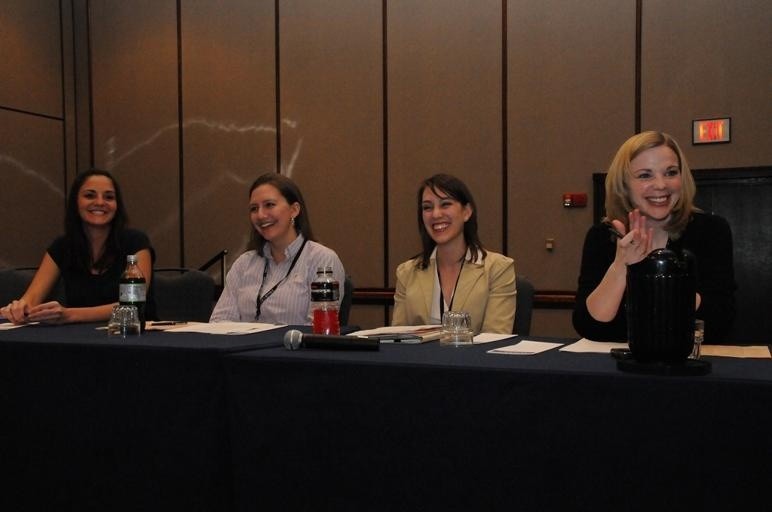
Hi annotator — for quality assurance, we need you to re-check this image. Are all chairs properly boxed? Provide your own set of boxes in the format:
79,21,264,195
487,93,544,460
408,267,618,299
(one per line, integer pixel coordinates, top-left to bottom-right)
341,275,353,326
150,268,217,321
0,266,58,306
513,275,534,335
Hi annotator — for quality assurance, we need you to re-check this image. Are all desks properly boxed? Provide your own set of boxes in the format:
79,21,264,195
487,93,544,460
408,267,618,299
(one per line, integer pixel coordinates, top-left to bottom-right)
0,320,772,512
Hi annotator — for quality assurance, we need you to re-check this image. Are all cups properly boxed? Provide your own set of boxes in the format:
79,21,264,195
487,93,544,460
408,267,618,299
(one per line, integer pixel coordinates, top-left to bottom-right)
439,311,474,348
107,305,141,338
688,319,704,359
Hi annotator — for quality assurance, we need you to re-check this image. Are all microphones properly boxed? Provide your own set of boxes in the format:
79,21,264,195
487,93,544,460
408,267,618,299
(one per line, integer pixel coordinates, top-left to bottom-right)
283,329,381,352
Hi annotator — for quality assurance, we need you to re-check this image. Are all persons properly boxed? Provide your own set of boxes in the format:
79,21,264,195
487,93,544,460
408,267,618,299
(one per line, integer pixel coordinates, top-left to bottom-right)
571,129,736,346
385,171,518,337
207,171,347,329
0,168,159,332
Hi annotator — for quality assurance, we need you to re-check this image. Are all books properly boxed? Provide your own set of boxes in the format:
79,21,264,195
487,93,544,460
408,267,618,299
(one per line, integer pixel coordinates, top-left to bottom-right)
342,323,450,346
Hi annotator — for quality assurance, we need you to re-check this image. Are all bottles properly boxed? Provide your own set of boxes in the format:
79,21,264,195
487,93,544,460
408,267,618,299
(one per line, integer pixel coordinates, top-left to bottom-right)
118,255,146,337
309,266,341,336
